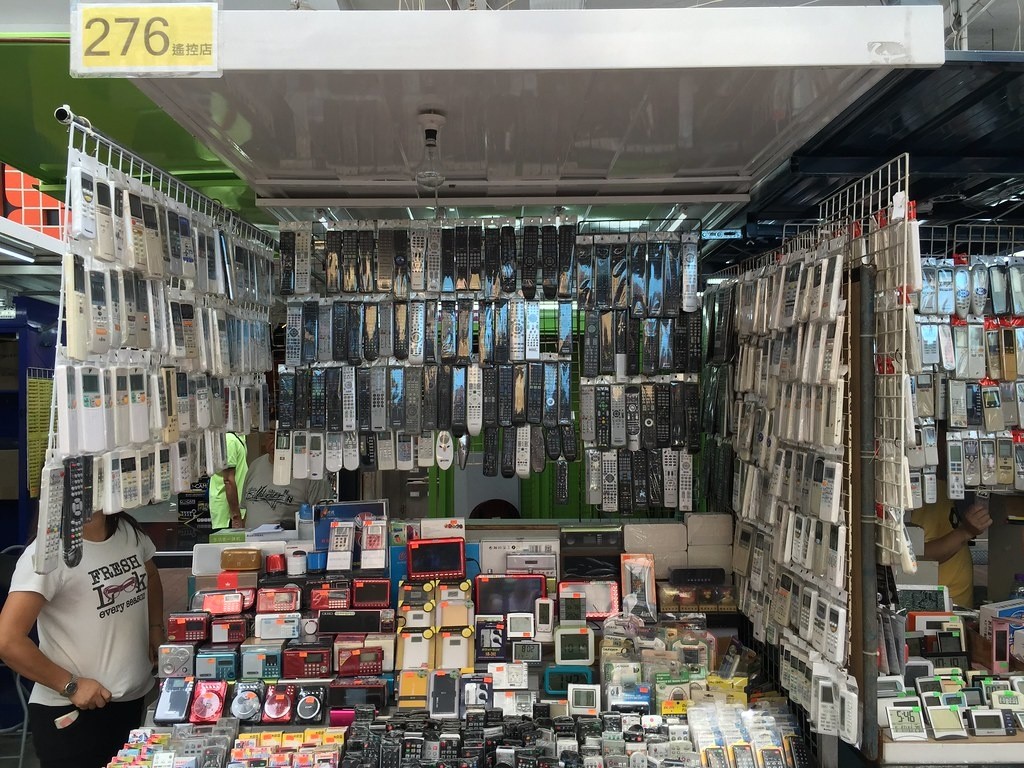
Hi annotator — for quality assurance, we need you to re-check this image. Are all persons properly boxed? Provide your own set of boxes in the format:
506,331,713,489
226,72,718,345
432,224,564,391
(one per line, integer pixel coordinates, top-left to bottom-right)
0,509,164,768
241,415,330,528
911,419,993,612
208,432,248,531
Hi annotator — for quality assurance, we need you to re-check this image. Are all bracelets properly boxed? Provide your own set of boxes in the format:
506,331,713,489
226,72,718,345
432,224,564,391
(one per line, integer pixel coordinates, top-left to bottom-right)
149,623,164,629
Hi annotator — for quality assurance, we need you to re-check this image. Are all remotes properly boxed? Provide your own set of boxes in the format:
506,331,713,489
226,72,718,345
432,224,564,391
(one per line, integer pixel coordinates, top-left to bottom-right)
36,170,704,565
853,228,1024,575
98,677,817,767
706,256,868,742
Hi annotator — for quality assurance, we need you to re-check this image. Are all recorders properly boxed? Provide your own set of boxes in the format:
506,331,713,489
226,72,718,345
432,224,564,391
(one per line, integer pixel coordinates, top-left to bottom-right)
158,519,716,713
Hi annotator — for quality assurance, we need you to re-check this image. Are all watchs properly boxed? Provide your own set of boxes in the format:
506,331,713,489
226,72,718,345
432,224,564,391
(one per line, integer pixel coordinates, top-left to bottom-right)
59,673,78,698
